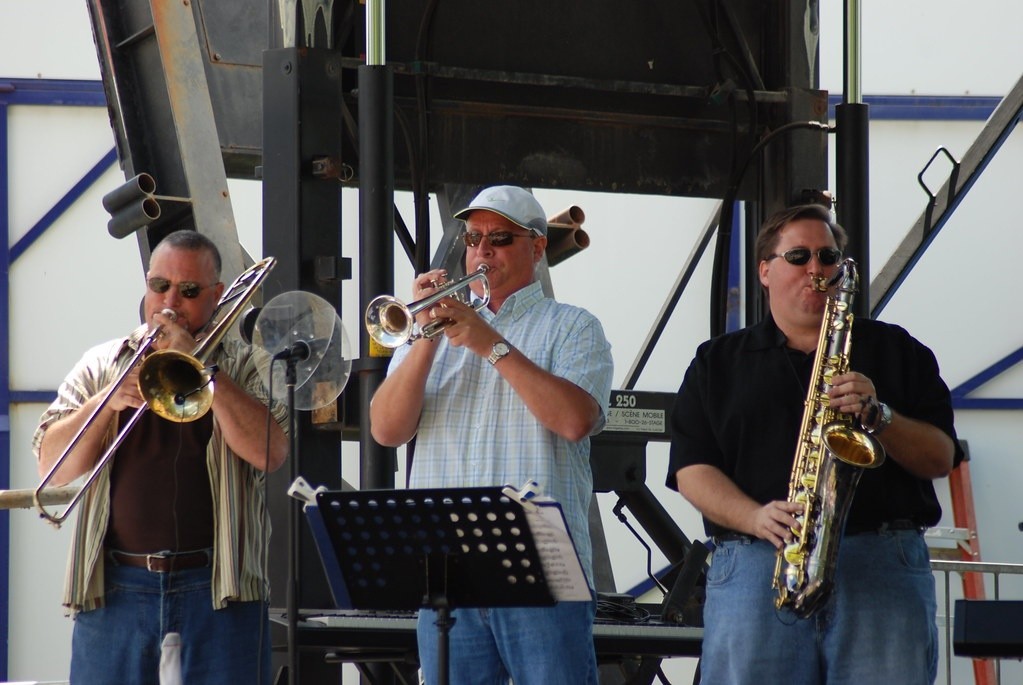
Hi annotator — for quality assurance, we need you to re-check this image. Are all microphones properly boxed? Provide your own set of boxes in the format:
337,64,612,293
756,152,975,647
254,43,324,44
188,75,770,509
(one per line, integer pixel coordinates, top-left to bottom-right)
274,340,311,362
174,392,186,405
865,405,878,426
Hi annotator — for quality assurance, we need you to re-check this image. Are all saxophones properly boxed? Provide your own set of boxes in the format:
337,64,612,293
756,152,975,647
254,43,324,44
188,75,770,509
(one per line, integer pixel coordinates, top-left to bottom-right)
768,256,886,620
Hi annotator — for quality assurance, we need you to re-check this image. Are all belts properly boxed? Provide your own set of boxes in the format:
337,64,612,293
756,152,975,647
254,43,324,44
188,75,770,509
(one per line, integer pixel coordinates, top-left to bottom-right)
105,548,215,573
714,521,916,541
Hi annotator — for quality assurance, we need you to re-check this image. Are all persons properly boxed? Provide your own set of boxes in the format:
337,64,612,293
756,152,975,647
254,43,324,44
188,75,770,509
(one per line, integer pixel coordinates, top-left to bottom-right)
369,185,614,685
32,231,297,685
665,205,962,685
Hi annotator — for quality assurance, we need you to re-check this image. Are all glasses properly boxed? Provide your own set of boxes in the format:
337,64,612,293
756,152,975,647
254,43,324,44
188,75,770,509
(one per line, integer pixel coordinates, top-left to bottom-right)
767,247,842,265
146,277,220,298
463,229,537,247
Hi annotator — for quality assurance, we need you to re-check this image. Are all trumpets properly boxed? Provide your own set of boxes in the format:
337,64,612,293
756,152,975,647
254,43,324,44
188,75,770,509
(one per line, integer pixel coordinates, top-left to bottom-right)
365,263,493,348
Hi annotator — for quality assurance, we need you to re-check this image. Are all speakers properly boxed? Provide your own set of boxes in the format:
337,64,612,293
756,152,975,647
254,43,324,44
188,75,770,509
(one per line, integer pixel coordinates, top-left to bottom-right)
953,599,1023,661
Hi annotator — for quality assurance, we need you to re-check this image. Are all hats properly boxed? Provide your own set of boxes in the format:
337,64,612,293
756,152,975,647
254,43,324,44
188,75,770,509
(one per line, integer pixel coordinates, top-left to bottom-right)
454,185,547,237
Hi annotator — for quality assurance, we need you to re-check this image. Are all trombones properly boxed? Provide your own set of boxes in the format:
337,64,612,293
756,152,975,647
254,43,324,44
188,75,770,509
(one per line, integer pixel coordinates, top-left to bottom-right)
33,257,276,529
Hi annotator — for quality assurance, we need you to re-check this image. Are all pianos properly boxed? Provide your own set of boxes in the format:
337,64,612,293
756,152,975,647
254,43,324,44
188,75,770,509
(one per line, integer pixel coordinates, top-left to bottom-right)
264,592,707,685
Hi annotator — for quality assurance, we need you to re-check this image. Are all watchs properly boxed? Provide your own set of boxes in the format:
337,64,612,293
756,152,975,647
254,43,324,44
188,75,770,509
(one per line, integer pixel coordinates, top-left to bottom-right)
487,339,512,366
862,402,893,434
